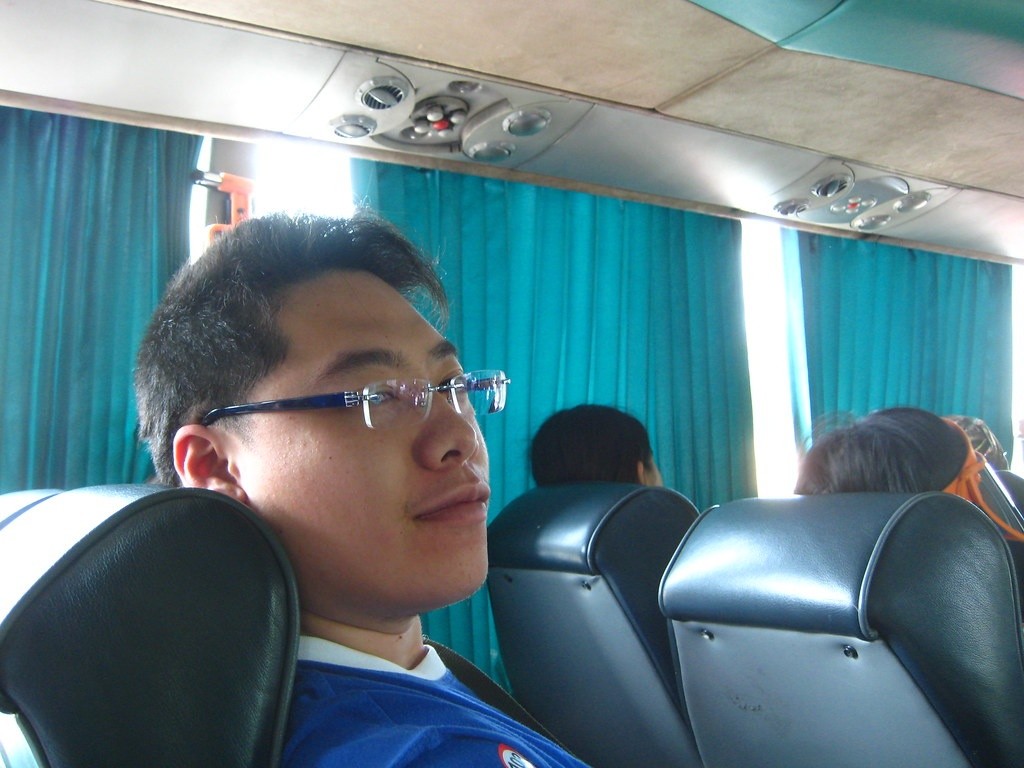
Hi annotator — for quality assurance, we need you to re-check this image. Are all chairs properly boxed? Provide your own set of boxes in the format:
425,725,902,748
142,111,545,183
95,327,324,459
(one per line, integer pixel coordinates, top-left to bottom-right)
658,492,1024,768
488,482,704,768
0,482,299,768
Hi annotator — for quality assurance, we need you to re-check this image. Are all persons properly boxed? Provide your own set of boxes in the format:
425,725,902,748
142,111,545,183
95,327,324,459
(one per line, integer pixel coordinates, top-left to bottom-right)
133,211,594,767
795,407,980,511
530,404,663,490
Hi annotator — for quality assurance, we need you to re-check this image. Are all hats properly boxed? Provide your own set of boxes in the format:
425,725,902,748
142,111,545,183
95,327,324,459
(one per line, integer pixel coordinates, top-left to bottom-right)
941,417,1024,542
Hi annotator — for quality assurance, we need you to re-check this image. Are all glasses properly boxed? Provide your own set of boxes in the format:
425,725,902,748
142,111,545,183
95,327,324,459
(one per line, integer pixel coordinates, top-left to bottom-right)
199,369,510,431
